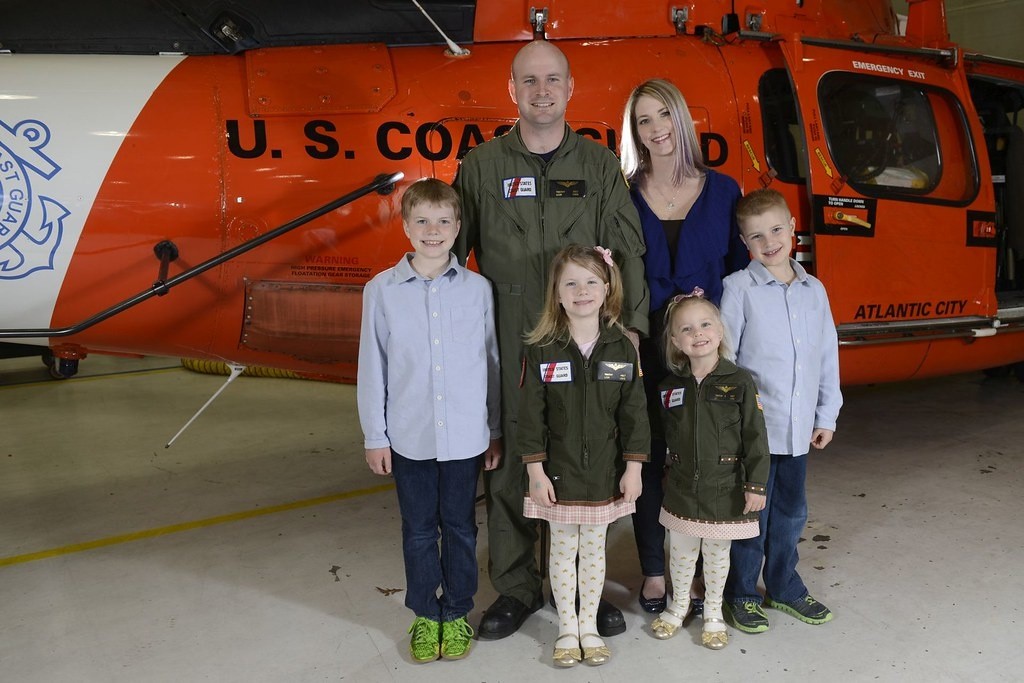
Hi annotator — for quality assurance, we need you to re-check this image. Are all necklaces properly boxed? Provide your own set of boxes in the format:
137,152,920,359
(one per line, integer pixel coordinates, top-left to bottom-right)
656,187,679,207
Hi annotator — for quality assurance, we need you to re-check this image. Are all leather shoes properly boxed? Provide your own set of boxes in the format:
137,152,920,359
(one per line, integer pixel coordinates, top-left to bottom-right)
478,594,530,639
639,578,666,612
690,583,706,614
550,590,626,637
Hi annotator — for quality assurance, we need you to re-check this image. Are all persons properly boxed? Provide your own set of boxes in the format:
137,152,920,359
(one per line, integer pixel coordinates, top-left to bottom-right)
647,287,770,650
514,243,650,667
450,41,646,638
622,81,751,616
721,190,843,633
357,177,501,662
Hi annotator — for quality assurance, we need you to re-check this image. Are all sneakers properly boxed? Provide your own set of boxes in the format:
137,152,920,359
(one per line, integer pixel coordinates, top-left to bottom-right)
764,593,833,624
408,616,441,663
441,614,474,659
723,601,768,632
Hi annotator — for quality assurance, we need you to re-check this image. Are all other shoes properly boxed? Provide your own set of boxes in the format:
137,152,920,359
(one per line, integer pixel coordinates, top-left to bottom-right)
701,618,728,649
552,634,581,666
652,604,694,640
580,634,610,665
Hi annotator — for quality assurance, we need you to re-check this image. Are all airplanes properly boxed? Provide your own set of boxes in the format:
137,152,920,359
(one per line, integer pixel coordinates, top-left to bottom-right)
2,0,1024,389
